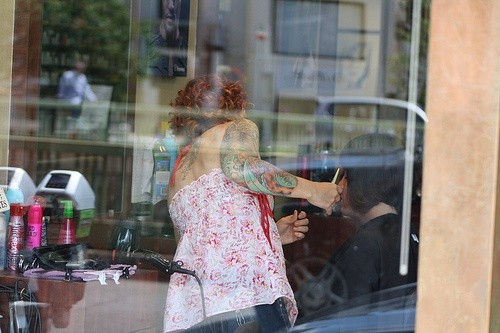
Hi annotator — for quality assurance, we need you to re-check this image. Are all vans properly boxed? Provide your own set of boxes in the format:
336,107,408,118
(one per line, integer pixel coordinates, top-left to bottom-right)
314,96,427,153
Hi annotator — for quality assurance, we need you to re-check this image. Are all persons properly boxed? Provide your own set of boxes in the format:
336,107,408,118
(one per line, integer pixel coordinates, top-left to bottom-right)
142,0,188,77
162,73,343,333
55,55,99,117
293,132,417,316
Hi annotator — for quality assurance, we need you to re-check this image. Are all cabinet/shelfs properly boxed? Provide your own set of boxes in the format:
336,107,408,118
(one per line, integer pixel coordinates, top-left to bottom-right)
33,0,136,218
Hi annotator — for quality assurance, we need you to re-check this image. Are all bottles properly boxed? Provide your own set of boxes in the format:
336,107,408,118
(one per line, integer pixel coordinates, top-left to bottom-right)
294,144,311,204
319,141,342,217
0,200,76,272
151,120,178,205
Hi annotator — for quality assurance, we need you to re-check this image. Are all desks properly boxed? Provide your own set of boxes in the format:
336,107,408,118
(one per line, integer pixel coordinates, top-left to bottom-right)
86,214,178,255
0,267,170,333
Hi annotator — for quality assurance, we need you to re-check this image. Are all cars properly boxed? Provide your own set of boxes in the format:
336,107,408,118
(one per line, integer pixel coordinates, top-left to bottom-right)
288,283,417,333
149,150,424,318
